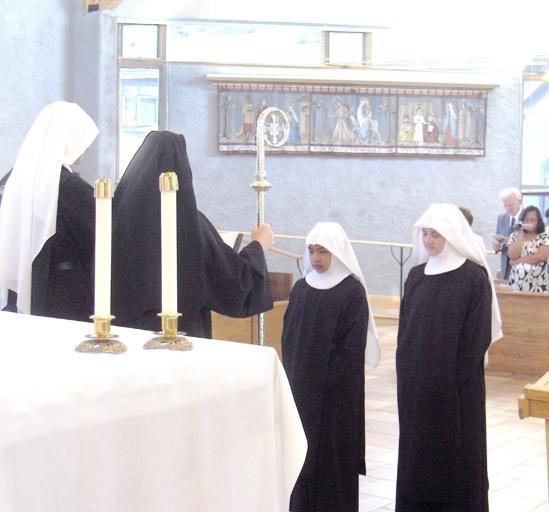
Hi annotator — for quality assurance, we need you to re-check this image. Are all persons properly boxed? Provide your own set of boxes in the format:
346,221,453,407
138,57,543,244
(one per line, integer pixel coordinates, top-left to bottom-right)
395,204,503,512
281,222,380,512
461,189,549,294
108,129,274,339
0,101,96,324
234,93,472,146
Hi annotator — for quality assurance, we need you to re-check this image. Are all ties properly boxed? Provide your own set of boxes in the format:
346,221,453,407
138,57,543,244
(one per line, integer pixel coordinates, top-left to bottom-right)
510,218,515,229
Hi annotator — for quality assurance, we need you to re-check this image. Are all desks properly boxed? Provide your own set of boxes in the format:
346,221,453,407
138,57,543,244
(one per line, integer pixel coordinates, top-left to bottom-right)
0,308,282,512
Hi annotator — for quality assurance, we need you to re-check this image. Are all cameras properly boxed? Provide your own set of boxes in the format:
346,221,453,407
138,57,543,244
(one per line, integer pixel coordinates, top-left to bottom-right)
517,221,533,231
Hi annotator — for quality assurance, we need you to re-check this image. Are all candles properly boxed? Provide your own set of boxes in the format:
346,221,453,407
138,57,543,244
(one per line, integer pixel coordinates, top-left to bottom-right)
158,172,180,315
91,178,117,320
